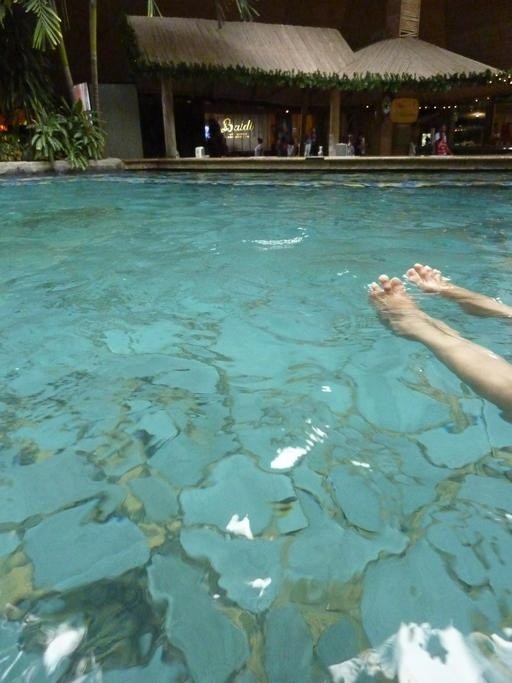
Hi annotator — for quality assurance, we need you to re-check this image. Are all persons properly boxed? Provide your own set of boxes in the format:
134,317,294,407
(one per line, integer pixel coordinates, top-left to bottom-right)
252,121,512,155
366,261,512,418
